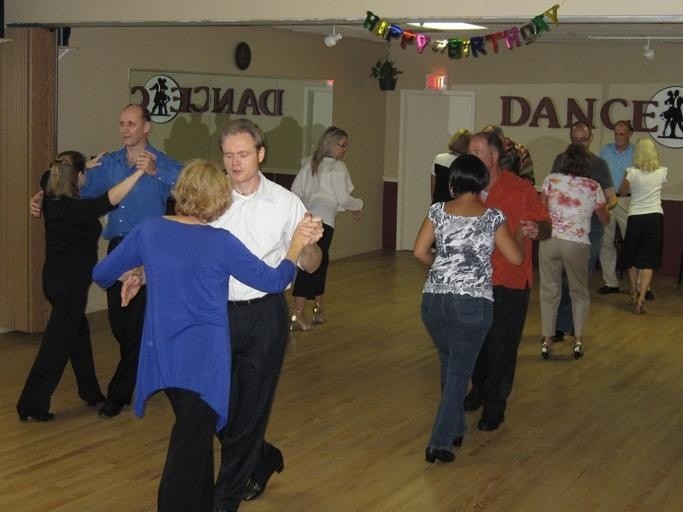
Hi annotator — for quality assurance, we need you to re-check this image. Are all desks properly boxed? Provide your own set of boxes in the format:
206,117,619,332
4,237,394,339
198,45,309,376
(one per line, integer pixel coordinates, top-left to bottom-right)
531,190,683,276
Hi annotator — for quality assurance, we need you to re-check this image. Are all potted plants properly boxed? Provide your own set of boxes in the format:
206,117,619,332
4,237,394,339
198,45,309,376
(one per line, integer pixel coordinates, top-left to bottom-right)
367,55,405,91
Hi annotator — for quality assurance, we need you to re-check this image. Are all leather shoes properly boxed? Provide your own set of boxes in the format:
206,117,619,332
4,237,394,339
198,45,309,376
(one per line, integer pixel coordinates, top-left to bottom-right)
241,442,283,501
98,401,132,418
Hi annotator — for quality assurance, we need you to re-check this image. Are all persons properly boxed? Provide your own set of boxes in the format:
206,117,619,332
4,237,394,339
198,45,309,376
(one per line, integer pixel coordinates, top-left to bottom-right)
463,132,553,431
29,103,189,418
287,125,365,331
17,149,158,422
92,158,324,512
412,153,532,463
430,127,476,205
120,116,327,512
481,124,535,186
540,121,668,359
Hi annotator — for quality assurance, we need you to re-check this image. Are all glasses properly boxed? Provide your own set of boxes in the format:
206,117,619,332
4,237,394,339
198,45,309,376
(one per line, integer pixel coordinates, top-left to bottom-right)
337,143,347,148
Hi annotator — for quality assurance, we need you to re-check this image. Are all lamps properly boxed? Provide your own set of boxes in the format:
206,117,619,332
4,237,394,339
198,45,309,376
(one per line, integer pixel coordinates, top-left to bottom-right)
643,39,656,61
324,26,343,47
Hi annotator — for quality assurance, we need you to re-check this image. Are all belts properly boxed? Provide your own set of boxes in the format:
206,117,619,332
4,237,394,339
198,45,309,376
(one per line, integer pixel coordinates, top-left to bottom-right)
228,294,270,306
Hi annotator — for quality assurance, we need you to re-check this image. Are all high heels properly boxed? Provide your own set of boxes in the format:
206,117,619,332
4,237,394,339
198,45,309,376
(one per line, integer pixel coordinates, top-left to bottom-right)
17,409,54,422
312,306,327,323
79,394,105,406
289,312,313,331
